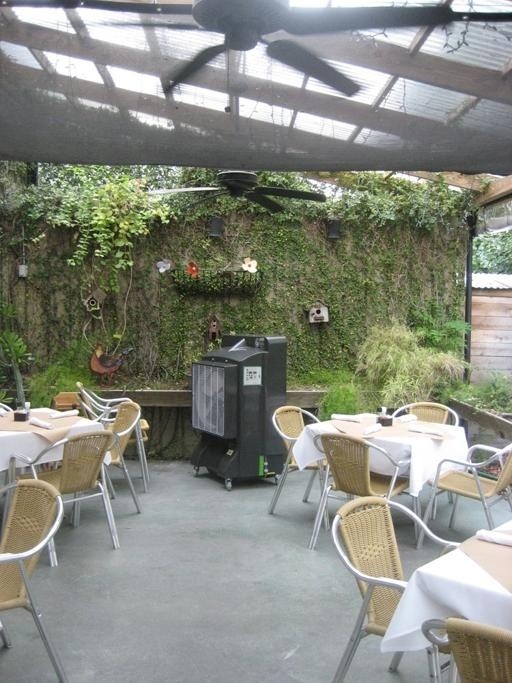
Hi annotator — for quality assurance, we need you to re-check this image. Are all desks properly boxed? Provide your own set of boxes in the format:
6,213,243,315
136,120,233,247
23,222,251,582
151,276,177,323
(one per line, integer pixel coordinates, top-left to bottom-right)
379,520,511,654
292,413,471,543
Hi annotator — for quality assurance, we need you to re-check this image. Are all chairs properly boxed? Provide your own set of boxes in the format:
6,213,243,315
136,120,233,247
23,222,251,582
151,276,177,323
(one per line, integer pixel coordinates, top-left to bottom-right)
416,442,511,549
0,403,13,416
311,433,411,554
14,430,117,567
330,496,463,683
81,402,142,515
271,406,328,532
75,381,151,483
392,404,459,520
76,395,148,493
0,478,65,683
421,615,511,683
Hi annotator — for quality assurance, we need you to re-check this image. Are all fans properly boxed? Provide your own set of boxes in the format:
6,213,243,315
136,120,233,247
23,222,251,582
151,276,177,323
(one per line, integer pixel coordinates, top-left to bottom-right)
146,170,331,214
79,0,453,96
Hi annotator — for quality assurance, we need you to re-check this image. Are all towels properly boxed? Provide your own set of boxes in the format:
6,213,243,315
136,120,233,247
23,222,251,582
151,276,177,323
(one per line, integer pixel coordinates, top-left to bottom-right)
0,408,7,417
30,418,54,429
50,409,79,418
